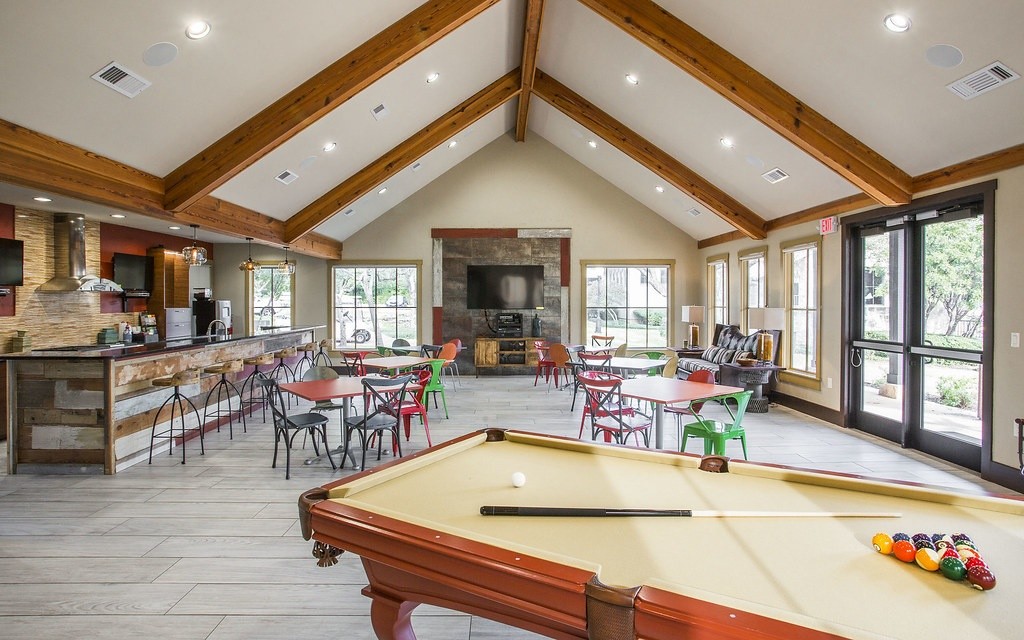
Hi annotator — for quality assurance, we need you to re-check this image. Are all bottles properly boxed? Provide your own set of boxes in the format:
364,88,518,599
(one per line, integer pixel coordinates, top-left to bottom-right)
532,314,541,337
123,322,132,342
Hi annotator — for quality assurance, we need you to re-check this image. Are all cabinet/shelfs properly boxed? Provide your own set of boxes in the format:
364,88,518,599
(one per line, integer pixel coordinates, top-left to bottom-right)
150,309,191,339
473,337,545,379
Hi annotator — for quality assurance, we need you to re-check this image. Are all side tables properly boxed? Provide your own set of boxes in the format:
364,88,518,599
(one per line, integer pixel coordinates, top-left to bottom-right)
723,361,786,414
667,346,704,375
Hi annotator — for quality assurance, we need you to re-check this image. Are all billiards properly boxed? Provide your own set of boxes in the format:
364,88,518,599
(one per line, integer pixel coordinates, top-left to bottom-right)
872,532,894,555
933,540,957,552
959,547,982,560
931,533,954,544
892,532,914,545
892,539,916,563
936,548,961,560
914,540,936,552
912,533,932,544
955,539,977,550
914,548,939,572
950,532,973,542
962,556,990,570
967,565,996,591
511,472,526,488
939,556,966,580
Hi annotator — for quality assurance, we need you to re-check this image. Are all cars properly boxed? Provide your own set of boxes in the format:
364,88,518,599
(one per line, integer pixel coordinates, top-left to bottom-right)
385,294,409,308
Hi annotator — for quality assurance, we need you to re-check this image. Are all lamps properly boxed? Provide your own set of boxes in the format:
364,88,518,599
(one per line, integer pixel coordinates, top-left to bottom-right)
682,304,705,349
182,225,207,267
749,307,785,366
239,238,262,271
278,247,296,273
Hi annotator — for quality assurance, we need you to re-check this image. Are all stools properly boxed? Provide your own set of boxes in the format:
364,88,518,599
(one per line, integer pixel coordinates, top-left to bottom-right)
148,339,334,465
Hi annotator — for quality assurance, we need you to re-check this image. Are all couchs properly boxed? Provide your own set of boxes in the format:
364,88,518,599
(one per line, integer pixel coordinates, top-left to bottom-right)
676,323,782,404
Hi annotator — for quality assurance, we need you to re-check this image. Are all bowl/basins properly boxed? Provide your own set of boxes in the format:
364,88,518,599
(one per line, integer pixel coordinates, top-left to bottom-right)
736,359,758,366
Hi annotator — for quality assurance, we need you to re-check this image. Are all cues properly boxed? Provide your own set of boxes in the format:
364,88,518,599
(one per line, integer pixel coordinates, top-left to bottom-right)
480,506,903,518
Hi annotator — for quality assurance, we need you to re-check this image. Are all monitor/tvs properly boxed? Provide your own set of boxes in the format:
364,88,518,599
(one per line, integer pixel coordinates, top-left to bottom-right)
0,238,24,286
114,251,155,291
466,265,544,309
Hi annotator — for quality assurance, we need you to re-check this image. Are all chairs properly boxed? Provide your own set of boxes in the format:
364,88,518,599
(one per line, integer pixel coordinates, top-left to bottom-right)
256,339,467,480
534,335,754,461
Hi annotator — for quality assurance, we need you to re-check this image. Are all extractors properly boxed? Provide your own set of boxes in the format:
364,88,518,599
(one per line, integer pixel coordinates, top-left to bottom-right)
34,214,123,290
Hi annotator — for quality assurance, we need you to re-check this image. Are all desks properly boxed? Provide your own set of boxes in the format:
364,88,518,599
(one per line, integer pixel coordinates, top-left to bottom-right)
297,429,1024,640
279,373,423,470
579,376,744,450
340,356,455,376
390,346,468,358
581,357,667,405
